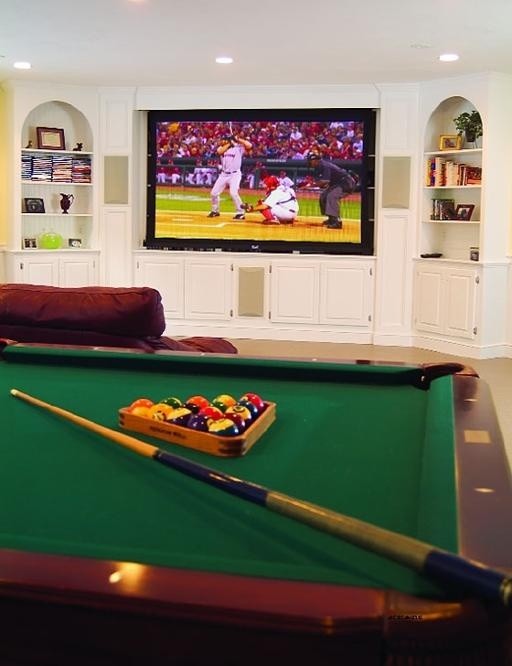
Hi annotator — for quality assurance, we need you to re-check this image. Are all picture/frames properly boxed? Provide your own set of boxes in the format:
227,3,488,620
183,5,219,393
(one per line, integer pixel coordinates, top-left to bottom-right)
35,125,67,150
24,195,46,213
67,237,83,247
23,236,39,250
451,201,476,221
438,133,461,150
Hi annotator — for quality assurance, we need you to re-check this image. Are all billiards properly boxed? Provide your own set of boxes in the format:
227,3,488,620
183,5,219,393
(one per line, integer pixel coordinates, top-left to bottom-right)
127,392,264,436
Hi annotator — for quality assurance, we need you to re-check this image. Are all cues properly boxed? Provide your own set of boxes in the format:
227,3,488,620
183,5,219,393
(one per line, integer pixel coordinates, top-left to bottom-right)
10,388,511,605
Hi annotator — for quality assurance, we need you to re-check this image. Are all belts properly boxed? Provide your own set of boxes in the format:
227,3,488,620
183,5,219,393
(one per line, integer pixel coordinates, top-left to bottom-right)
222,170,239,175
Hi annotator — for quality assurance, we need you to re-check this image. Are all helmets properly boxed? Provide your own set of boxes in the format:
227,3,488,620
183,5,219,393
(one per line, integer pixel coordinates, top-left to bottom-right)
220,131,231,140
263,176,279,192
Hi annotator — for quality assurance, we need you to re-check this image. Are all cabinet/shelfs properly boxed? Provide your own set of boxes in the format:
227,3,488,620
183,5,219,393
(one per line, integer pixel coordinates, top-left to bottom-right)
98,84,142,287
132,250,233,328
233,253,269,329
422,147,481,230
269,251,372,327
376,82,419,345
2,246,99,289
410,253,511,362
18,145,94,217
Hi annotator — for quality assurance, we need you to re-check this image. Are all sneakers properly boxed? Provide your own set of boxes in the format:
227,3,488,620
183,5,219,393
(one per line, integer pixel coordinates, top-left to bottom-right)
323,219,342,230
262,218,281,225
207,213,220,217
233,215,245,220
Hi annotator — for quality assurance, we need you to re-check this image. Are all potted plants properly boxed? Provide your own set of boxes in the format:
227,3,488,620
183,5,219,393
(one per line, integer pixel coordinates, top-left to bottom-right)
452,108,483,143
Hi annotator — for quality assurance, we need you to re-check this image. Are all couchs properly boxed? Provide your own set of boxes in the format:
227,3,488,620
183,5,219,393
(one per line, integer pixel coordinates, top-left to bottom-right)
1,281,240,355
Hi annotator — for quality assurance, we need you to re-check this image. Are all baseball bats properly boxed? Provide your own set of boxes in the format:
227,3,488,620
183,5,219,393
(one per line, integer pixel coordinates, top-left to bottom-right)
229,121,233,134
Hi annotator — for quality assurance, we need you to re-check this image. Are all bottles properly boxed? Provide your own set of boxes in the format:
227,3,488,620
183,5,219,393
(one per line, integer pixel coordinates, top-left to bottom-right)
470,246,479,261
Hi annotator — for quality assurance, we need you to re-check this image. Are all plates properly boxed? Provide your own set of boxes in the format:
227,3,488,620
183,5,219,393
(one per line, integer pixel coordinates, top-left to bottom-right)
420,253,442,258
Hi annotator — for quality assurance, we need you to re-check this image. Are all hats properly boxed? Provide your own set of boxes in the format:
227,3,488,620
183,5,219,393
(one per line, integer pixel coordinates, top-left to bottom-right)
308,154,319,161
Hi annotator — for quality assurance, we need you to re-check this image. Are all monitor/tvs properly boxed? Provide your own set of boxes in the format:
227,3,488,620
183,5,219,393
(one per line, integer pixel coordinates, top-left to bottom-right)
146,109,376,257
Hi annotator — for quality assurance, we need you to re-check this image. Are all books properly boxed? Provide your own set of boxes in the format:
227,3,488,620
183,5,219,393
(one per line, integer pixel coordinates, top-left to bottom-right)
426,156,481,187
21,154,91,183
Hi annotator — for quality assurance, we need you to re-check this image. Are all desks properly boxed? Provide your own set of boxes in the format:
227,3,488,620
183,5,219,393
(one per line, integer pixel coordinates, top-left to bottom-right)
1,332,511,665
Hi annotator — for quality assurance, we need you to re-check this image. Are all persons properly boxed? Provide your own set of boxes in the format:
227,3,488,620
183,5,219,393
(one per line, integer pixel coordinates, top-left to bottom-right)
156,121,364,228
